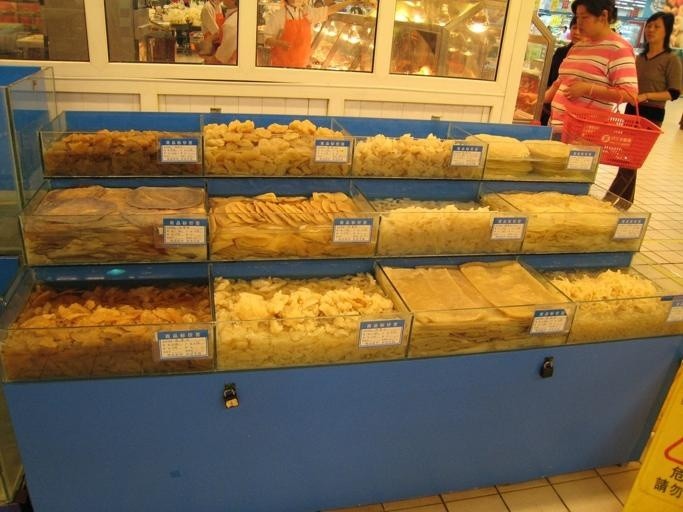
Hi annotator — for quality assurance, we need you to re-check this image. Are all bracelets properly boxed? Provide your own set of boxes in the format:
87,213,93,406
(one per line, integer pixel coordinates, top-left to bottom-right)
588,83,594,98
642,92,648,102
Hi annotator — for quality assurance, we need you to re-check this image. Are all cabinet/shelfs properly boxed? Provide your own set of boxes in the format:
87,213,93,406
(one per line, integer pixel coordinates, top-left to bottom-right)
310,0,556,130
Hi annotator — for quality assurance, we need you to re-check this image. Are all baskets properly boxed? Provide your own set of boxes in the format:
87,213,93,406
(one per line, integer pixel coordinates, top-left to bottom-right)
561,86,663,170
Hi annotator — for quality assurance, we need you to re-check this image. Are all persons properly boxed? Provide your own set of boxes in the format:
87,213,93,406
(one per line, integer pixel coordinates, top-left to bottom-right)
258,1,369,68
199,0,224,40
558,27,567,40
193,0,237,65
602,12,682,210
540,16,583,126
526,1,639,202
